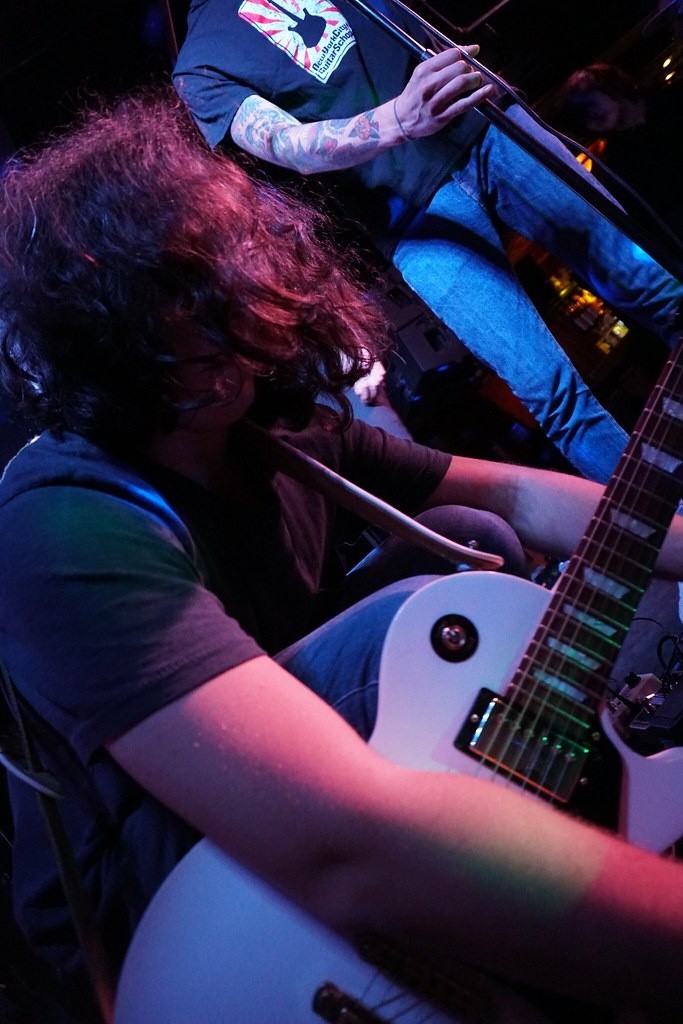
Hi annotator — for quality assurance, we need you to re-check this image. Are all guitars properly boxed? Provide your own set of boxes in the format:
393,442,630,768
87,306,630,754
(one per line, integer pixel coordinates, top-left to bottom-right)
109,342,683,1024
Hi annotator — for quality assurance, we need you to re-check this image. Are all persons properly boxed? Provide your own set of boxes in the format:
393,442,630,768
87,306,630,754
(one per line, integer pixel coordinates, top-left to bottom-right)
0,83,683,1024
171,0,683,516
555,63,658,136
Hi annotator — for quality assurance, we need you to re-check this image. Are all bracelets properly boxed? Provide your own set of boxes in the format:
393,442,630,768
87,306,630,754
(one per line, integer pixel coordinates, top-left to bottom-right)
393,98,417,140
